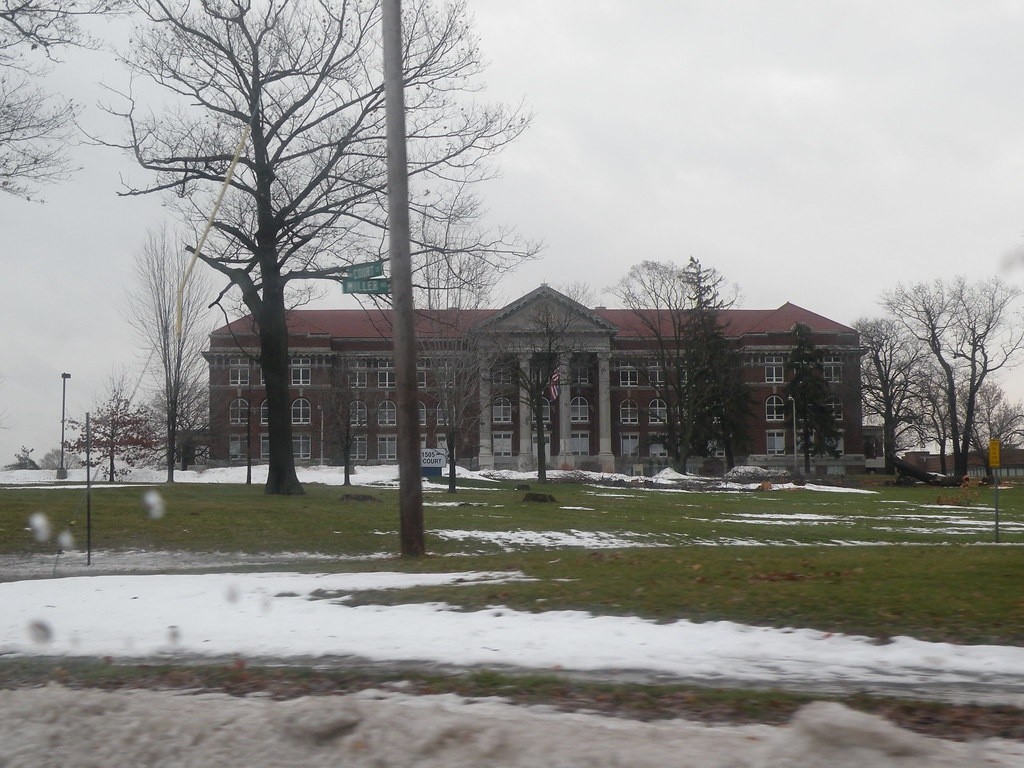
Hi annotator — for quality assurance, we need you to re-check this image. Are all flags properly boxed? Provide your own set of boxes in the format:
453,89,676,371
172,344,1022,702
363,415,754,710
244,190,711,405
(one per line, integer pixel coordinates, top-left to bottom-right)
549,362,561,401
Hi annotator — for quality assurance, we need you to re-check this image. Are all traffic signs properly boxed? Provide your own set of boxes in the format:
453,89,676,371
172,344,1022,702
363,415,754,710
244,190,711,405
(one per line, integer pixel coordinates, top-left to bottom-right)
348,263,384,279
343,279,388,294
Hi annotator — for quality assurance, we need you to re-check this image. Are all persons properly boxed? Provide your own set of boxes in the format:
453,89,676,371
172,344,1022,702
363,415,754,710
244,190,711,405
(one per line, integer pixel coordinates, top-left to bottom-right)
962,473,969,488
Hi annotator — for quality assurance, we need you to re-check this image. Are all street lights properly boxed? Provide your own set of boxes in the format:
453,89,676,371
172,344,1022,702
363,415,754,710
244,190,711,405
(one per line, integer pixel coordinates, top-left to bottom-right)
57,372,71,477
789,396,797,470
